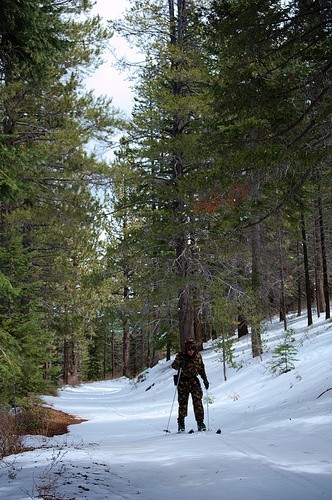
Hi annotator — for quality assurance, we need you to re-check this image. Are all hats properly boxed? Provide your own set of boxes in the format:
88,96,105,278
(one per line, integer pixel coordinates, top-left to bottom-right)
185,339,197,347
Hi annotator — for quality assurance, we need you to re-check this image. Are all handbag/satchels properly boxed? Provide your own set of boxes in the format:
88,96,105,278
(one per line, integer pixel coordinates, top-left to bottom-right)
173,375,178,386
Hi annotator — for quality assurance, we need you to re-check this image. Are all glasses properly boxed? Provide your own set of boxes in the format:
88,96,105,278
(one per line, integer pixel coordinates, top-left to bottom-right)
188,348,194,351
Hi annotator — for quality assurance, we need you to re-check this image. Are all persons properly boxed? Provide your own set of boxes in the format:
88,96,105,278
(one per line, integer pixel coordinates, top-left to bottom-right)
171,339,209,433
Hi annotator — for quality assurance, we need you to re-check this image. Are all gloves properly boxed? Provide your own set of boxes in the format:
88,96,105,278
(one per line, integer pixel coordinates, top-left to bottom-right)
204,379,209,390
178,361,184,368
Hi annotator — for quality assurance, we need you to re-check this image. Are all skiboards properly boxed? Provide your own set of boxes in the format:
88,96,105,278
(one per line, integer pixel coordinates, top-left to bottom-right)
177,429,194,434
195,429,222,434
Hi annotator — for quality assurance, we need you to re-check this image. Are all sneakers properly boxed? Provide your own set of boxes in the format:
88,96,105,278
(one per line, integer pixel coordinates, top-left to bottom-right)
197,422,206,430
177,422,185,431
